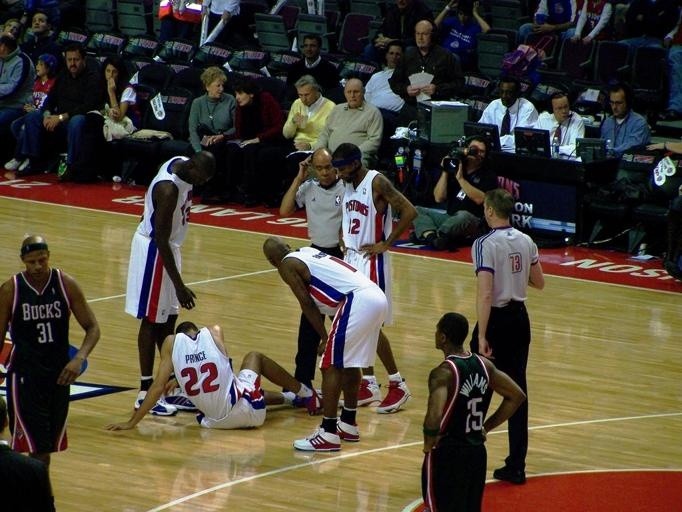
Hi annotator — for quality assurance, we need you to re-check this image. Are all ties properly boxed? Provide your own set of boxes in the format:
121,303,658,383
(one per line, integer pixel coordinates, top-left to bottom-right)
500,109,510,136
553,123,561,144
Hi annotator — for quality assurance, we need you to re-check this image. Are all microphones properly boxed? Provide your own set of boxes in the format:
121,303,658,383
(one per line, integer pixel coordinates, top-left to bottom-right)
567,110,573,119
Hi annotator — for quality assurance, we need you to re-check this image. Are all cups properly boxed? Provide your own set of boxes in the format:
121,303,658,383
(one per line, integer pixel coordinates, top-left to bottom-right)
637,244,646,256
298,116,309,130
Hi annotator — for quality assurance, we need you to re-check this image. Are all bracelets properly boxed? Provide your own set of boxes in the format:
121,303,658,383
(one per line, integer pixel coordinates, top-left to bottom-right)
480,425,487,436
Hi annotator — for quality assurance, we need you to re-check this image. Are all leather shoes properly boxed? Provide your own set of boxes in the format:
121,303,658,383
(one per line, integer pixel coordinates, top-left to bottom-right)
15,164,48,177
659,111,682,121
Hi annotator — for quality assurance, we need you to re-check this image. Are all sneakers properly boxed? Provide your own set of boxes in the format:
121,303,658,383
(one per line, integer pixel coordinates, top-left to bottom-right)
409,232,445,251
4,158,29,171
293,428,342,451
135,388,198,415
292,378,381,416
494,466,525,483
336,417,360,441
378,378,410,414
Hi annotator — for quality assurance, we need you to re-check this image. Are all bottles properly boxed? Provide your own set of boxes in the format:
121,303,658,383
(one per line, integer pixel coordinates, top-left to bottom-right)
605,140,613,161
552,137,559,159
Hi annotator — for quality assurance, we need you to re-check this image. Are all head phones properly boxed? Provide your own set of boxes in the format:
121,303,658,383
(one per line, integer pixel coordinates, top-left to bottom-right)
415,16,439,43
603,83,631,110
546,91,572,114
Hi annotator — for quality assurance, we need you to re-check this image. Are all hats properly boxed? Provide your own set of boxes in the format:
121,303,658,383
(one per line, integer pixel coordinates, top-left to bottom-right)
39,54,56,66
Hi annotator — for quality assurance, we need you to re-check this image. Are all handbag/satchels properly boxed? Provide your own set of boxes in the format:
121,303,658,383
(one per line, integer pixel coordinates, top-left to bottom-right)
501,44,546,84
103,116,134,140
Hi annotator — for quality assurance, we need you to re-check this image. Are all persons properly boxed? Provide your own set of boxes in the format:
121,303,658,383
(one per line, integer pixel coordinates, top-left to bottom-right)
261,236,389,453
277,146,345,397
468,187,546,484
123,150,216,417
0,397,58,512
0,0,681,283
1,234,100,467
331,143,421,416
419,310,526,512
103,320,324,433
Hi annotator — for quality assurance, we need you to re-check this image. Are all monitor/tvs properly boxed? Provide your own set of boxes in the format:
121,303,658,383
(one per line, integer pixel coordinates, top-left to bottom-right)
463,122,501,151
514,127,551,158
575,138,606,161
